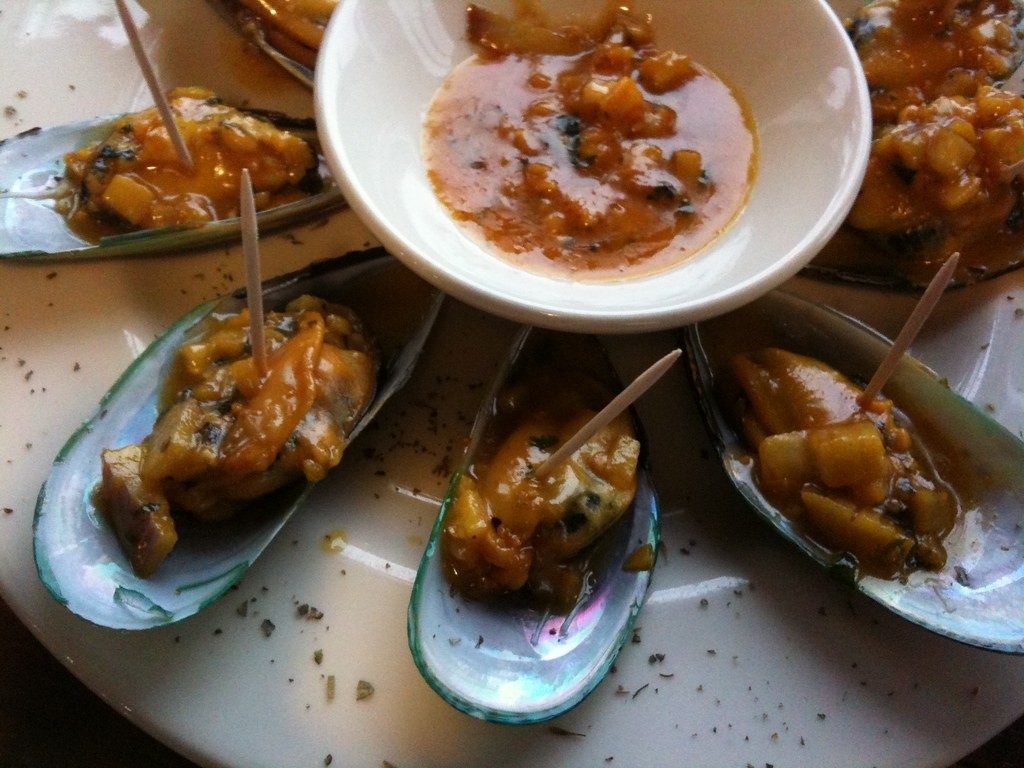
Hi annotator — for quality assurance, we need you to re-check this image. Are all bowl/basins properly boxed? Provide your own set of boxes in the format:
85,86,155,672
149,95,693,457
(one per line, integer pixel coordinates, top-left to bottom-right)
313,0,874,336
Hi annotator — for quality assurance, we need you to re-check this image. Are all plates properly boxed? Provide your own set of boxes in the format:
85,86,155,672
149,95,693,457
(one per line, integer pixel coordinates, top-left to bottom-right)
0,0,1024,768
